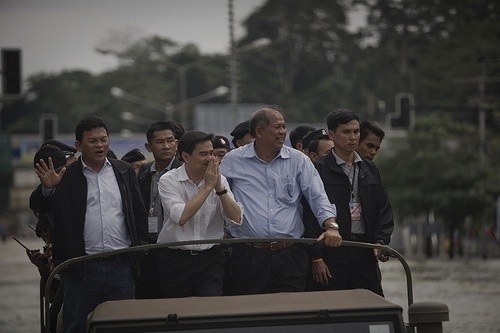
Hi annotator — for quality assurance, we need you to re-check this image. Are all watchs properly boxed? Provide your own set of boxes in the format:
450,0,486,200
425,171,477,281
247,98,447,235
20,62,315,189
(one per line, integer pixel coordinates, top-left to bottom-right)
327,223,340,230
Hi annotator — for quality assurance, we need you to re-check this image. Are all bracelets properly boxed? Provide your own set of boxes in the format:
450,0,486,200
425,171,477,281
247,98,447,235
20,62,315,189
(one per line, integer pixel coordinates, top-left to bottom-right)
312,258,322,263
217,187,228,196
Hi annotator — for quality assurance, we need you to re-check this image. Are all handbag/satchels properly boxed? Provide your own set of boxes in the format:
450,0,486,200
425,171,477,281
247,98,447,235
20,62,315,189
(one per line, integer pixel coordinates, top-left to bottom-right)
132,247,160,285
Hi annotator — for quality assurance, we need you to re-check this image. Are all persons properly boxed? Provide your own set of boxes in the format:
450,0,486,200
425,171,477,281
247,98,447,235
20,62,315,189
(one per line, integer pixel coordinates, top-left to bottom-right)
25,116,146,303
138,107,394,299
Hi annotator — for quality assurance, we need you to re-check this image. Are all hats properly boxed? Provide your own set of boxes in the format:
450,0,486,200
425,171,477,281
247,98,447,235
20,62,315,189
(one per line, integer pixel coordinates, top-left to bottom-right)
208,134,230,149
231,119,250,134
121,148,147,161
302,127,329,147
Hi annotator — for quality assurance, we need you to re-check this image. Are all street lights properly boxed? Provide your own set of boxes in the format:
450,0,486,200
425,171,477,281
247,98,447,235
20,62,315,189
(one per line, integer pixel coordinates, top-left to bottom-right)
108,84,231,122
95,37,272,139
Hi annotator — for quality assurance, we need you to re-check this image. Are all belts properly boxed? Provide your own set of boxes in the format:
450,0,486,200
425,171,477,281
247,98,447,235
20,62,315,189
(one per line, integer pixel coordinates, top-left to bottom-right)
176,247,218,256
245,240,295,249
92,254,117,260
